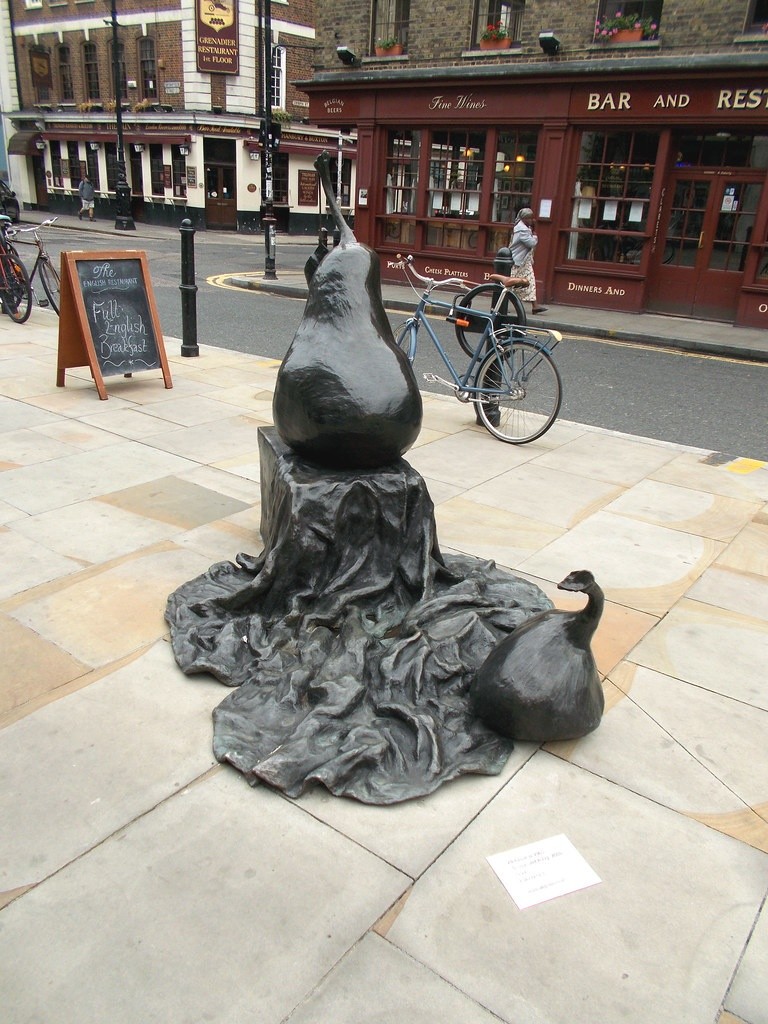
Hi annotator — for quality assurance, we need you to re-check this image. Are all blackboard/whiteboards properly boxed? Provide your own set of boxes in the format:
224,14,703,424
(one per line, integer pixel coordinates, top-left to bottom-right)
56,250,173,401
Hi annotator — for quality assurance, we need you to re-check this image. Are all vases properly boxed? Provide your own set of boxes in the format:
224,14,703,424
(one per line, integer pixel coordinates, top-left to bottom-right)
609,28,644,42
480,37,513,50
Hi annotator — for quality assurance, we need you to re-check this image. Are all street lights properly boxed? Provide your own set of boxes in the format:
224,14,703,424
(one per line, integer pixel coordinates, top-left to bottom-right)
103,0,137,230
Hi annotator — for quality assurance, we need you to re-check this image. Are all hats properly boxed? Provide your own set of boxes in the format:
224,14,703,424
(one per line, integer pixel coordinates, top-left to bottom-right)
514,208,534,226
85,175,93,181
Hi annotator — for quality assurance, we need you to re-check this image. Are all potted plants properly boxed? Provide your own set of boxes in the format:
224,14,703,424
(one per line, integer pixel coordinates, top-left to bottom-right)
374,36,404,57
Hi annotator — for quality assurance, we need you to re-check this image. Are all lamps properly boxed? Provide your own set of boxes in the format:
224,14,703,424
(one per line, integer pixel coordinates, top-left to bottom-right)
134,140,147,153
35,138,47,152
178,137,197,156
90,140,102,151
538,32,560,56
337,46,357,65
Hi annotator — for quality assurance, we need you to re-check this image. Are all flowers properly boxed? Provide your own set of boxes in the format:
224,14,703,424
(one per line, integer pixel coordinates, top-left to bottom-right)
594,11,657,49
480,20,510,41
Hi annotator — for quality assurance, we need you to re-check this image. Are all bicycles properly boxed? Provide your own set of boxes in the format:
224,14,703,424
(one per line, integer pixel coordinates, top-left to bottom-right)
0,213,50,324
6,217,60,316
392,254,563,444
584,213,674,265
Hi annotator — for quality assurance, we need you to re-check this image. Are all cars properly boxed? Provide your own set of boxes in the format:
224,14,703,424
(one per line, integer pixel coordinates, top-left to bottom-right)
0,179,20,224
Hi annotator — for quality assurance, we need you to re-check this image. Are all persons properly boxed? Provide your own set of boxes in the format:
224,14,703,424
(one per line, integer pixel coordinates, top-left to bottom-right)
508,208,548,314
78,175,96,222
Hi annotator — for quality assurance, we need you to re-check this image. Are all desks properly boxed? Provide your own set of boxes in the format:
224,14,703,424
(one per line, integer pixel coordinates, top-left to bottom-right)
65,188,80,202
94,190,101,203
145,195,166,211
47,186,65,200
100,191,124,205
167,197,188,212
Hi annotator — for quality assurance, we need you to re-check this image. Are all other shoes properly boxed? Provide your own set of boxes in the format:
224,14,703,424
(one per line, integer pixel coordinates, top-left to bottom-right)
78,210,83,220
532,307,549,315
90,217,96,222
